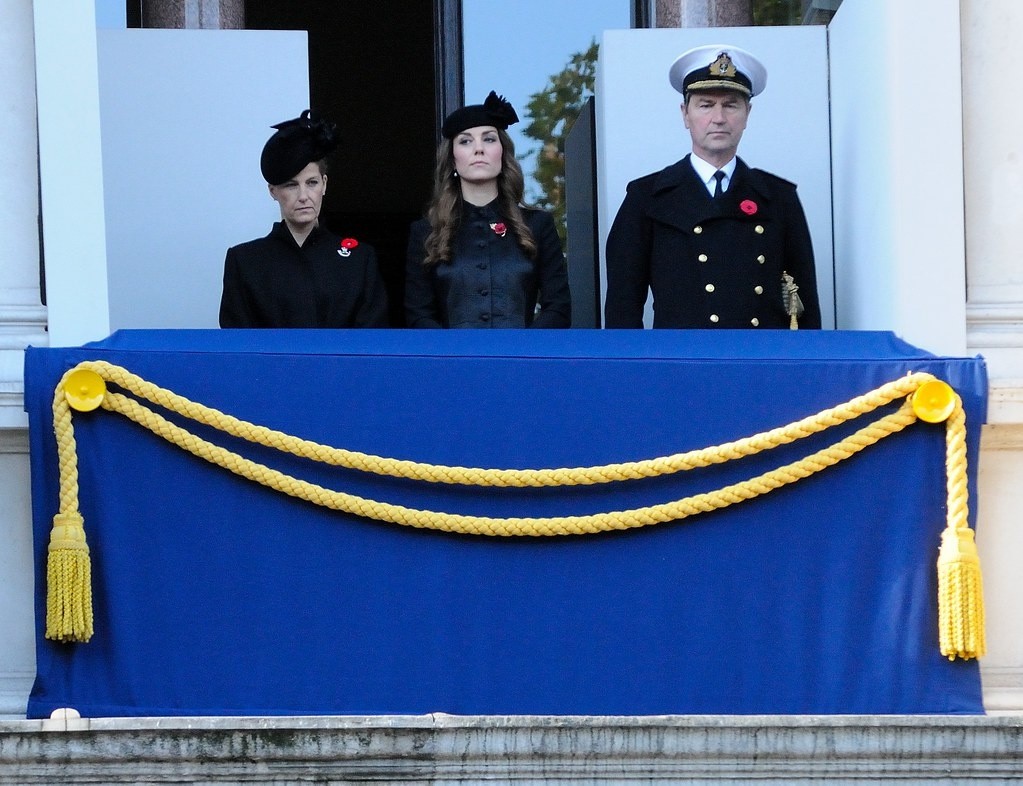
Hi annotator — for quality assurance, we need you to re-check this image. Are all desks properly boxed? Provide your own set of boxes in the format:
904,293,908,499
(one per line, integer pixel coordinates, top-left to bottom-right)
22,328,989,719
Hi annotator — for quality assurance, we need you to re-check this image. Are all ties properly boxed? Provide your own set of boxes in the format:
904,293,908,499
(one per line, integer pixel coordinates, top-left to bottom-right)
712,170,727,207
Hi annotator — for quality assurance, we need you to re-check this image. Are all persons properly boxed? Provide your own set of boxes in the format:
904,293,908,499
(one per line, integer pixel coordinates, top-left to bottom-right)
603,45,823,330
218,110,391,329
405,90,572,329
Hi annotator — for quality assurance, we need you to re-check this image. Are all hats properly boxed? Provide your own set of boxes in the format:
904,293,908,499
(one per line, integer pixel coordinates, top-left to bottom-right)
442,90,520,140
259,108,338,185
668,43,768,101
